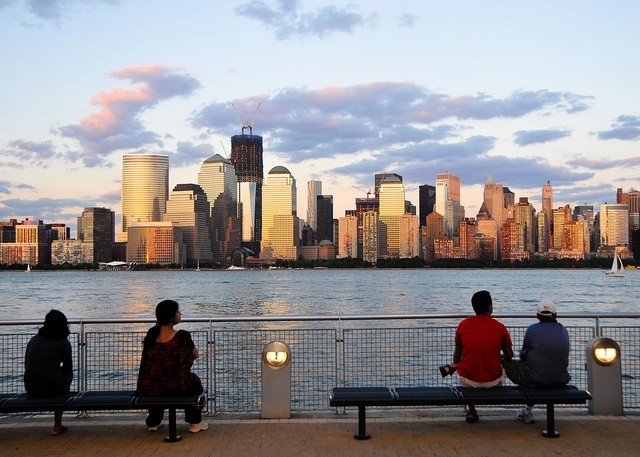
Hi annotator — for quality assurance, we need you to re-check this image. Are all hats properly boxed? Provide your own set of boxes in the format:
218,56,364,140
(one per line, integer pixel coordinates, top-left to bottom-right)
535,300,555,316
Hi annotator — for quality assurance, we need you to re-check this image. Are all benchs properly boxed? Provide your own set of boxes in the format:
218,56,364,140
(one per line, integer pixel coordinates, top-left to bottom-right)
2,388,208,442
329,385,593,441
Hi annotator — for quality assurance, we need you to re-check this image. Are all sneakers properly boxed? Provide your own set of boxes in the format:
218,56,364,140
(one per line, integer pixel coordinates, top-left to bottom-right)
517,409,533,423
188,420,208,433
149,420,163,431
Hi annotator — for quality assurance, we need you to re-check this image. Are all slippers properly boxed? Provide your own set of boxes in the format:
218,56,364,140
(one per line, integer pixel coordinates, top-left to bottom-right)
51,425,68,436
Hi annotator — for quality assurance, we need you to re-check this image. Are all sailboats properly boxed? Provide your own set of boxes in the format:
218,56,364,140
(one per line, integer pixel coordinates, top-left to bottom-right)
605,250,626,276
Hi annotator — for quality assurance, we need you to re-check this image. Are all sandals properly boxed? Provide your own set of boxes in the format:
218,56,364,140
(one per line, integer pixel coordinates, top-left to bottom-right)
439,365,452,377
466,412,479,423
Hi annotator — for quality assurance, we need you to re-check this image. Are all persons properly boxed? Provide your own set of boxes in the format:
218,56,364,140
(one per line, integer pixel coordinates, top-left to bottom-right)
439,290,514,424
23,309,73,436
500,300,571,424
137,299,209,433
